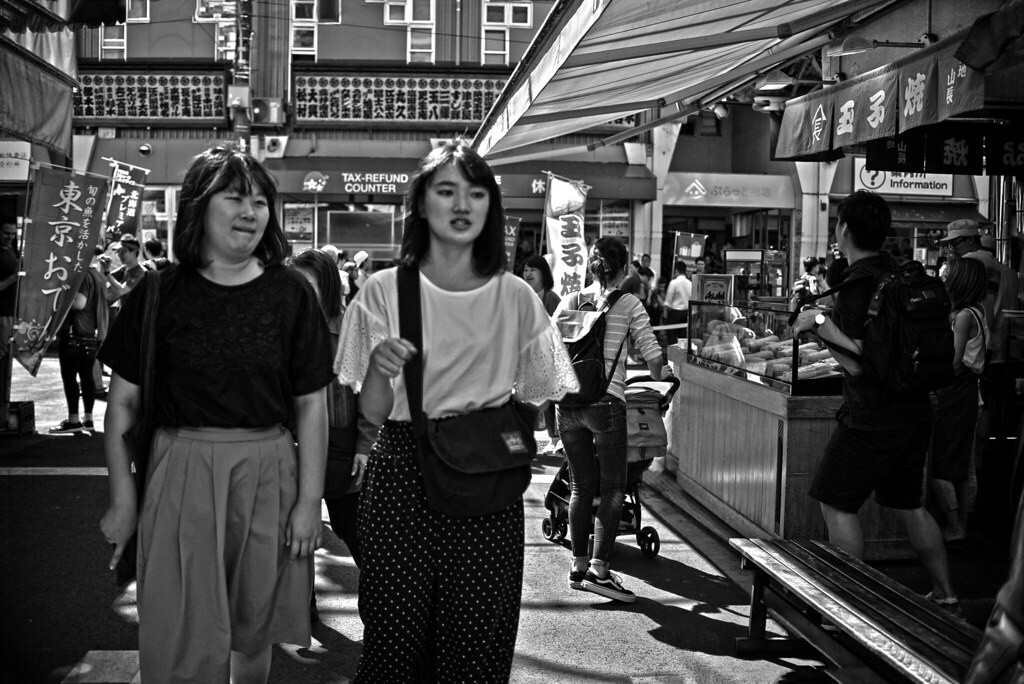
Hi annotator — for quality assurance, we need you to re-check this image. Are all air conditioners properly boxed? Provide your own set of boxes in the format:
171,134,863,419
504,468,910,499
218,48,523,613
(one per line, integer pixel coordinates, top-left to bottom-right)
250,97,284,125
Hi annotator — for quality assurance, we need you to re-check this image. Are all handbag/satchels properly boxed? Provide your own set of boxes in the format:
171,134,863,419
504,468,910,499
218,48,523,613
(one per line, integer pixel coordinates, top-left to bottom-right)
71,338,99,357
111,410,156,586
396,261,537,513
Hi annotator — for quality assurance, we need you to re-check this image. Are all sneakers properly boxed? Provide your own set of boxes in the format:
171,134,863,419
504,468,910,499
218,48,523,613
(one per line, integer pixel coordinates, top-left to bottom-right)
570,566,590,588
580,571,636,603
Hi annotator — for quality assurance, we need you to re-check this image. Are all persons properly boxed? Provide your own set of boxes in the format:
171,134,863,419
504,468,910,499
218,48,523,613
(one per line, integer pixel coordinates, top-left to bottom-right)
49,234,171,433
787,187,962,630
692,257,710,273
0,217,21,434
618,252,658,326
328,142,579,684
283,242,379,622
932,214,1024,541
663,261,692,345
97,145,335,684
550,236,673,602
792,255,839,307
521,254,561,316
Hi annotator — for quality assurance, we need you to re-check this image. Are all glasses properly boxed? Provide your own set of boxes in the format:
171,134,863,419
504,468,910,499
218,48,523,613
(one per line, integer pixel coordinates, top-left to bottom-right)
948,238,968,252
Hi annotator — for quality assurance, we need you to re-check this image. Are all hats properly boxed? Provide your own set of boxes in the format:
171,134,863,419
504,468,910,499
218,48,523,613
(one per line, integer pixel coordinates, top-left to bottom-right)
353,251,369,267
939,218,979,242
981,235,996,250
112,241,138,251
321,245,338,264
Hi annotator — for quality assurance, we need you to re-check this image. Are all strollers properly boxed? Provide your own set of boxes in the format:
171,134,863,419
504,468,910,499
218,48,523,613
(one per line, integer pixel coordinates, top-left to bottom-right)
541,376,682,557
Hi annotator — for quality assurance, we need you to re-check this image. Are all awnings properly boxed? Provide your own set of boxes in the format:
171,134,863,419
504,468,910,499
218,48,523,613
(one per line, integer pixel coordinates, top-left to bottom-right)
468,0,904,170
261,156,657,204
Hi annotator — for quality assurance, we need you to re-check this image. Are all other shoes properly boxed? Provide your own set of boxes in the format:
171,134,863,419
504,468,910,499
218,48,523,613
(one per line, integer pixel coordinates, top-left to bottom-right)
48,420,82,433
81,421,94,431
925,592,962,615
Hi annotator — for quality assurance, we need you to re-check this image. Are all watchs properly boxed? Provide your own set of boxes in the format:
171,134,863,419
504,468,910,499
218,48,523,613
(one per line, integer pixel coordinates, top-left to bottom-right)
812,311,829,332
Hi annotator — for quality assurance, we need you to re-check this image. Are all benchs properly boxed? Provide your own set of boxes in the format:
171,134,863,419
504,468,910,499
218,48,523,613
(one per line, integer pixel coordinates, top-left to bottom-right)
728,536,984,684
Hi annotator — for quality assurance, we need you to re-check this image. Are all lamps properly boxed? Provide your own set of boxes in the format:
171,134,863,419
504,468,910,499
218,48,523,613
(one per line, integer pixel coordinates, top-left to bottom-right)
826,33,939,59
754,68,843,88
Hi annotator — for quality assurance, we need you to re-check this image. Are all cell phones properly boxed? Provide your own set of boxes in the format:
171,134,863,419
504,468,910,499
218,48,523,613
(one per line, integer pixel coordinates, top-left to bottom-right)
111,533,140,588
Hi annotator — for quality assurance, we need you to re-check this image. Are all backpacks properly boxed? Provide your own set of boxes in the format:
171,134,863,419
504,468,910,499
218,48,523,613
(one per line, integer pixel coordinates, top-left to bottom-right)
555,290,631,407
797,257,956,424
